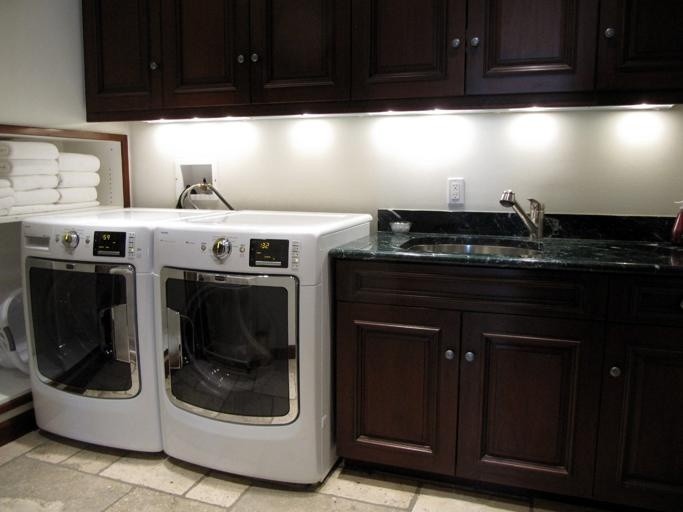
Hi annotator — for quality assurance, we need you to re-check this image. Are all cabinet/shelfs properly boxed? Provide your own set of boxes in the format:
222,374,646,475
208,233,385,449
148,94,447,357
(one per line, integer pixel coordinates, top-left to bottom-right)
161,0,349,119
593,267,683,512
0,124,130,447
349,0,599,113
333,255,614,512
599,0,683,105
81,0,161,123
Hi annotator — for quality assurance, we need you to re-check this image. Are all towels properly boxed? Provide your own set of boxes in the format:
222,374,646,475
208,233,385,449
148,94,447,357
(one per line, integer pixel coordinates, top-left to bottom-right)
0,140,101,215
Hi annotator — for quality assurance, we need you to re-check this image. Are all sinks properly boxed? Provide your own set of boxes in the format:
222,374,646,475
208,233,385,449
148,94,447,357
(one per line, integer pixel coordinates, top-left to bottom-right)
398,235,545,257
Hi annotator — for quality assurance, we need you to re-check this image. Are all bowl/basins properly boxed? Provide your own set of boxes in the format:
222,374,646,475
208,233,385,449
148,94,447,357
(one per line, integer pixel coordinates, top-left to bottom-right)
390,221,411,234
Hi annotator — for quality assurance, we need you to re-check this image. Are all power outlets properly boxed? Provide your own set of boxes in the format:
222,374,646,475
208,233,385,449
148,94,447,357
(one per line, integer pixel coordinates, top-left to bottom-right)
448,178,465,205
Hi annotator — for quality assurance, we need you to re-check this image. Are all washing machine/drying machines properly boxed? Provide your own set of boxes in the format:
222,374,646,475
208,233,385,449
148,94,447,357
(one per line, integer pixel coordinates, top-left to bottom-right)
154,209,373,486
20,207,236,452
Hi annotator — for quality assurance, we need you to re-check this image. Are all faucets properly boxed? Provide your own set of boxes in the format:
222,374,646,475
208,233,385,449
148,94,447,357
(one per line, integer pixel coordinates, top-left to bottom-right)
499,189,544,240
